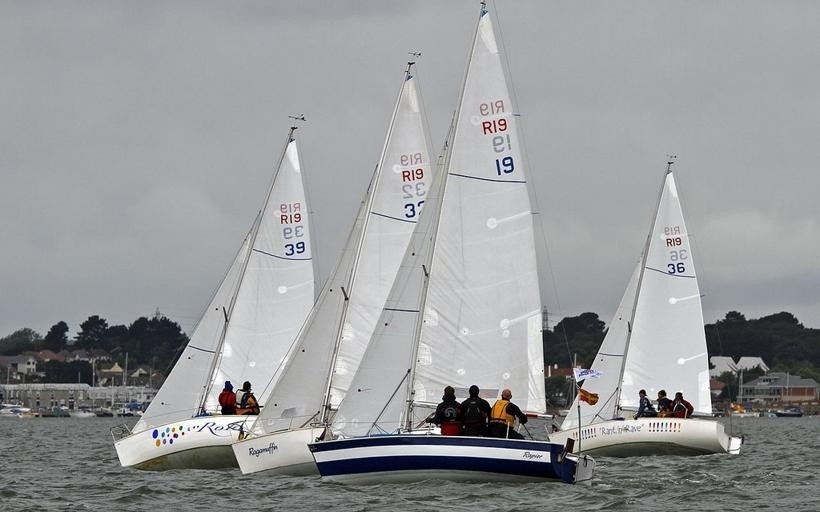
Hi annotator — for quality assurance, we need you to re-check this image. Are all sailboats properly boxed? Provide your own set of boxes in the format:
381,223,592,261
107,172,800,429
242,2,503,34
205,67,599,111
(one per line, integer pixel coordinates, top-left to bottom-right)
229,45,445,478
544,149,751,462
115,110,328,480
303,0,599,489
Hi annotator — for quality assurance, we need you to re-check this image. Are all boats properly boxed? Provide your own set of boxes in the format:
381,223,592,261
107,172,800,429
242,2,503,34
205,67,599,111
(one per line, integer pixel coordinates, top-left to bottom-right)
774,406,804,418
0,401,146,421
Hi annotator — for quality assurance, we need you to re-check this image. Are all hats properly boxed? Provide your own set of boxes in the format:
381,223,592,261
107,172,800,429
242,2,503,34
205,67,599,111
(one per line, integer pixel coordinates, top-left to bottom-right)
639,390,647,396
659,390,666,396
225,381,233,390
502,389,511,399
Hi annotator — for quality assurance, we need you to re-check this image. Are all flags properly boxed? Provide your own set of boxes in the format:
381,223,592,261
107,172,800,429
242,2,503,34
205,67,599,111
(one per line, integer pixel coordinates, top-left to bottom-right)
579,388,599,405
573,367,603,383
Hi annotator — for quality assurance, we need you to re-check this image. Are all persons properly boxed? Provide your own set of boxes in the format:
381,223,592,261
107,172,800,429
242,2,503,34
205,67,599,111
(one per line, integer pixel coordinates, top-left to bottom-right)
434,386,464,436
637,390,656,417
487,389,527,440
460,385,492,436
219,381,236,415
657,390,672,413
234,381,260,415
670,392,693,418
